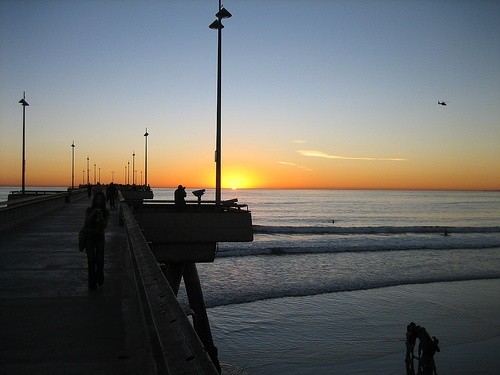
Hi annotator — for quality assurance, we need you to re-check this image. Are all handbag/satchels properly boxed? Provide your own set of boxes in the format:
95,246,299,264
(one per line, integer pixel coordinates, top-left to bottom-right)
79,227,86,252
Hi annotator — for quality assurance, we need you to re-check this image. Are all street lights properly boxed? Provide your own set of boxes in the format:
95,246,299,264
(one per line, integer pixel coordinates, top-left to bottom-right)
71,127,149,189
208,0,232,205
18,91,29,194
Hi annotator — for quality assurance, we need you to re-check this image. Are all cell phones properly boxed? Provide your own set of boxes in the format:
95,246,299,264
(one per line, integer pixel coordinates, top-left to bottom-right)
95,209,102,213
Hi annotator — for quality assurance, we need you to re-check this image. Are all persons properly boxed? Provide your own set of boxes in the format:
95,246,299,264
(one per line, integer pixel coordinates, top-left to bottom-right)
416,325,435,368
84,191,110,292
86,182,151,209
174,185,187,205
405,322,417,361
